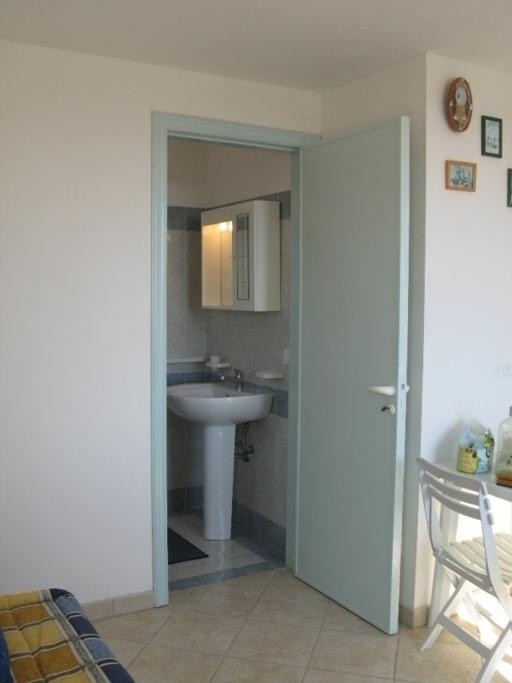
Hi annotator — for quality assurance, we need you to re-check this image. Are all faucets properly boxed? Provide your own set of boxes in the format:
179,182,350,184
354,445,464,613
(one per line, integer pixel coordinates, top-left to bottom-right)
216,369,244,393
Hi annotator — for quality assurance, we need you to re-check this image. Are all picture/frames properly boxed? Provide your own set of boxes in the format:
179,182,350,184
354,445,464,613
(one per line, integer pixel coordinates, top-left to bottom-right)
445,160,477,192
446,77,473,132
481,115,503,159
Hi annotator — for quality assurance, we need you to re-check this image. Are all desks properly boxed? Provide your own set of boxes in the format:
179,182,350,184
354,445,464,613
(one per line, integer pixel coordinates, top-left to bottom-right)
427,452,512,631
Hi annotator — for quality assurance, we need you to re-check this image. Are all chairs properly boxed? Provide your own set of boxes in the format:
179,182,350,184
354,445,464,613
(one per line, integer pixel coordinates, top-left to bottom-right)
415,455,512,683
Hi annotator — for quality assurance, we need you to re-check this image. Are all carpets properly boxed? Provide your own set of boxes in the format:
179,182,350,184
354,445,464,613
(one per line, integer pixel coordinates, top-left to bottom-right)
168,526,209,564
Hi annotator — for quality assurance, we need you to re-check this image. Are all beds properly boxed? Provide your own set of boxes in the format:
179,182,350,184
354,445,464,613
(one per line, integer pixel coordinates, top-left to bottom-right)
0,588,136,683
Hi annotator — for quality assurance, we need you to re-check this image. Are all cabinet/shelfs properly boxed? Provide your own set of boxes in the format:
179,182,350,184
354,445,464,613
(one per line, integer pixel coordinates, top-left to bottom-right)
200,199,281,312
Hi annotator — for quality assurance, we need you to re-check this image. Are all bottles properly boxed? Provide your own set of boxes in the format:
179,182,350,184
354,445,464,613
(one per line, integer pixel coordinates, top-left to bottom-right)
495,405,512,488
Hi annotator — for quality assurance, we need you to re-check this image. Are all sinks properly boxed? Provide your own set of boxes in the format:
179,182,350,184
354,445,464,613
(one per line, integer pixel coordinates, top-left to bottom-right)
166,381,275,424
